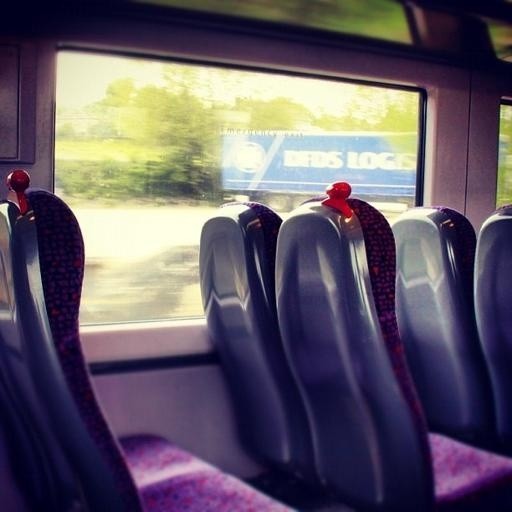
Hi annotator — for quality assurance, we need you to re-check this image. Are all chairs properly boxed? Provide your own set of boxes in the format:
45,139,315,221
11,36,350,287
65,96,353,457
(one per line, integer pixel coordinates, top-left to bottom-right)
1,168,298,510
115,422,214,490
386,205,479,451
471,203,510,458
274,180,510,511
194,200,282,511
